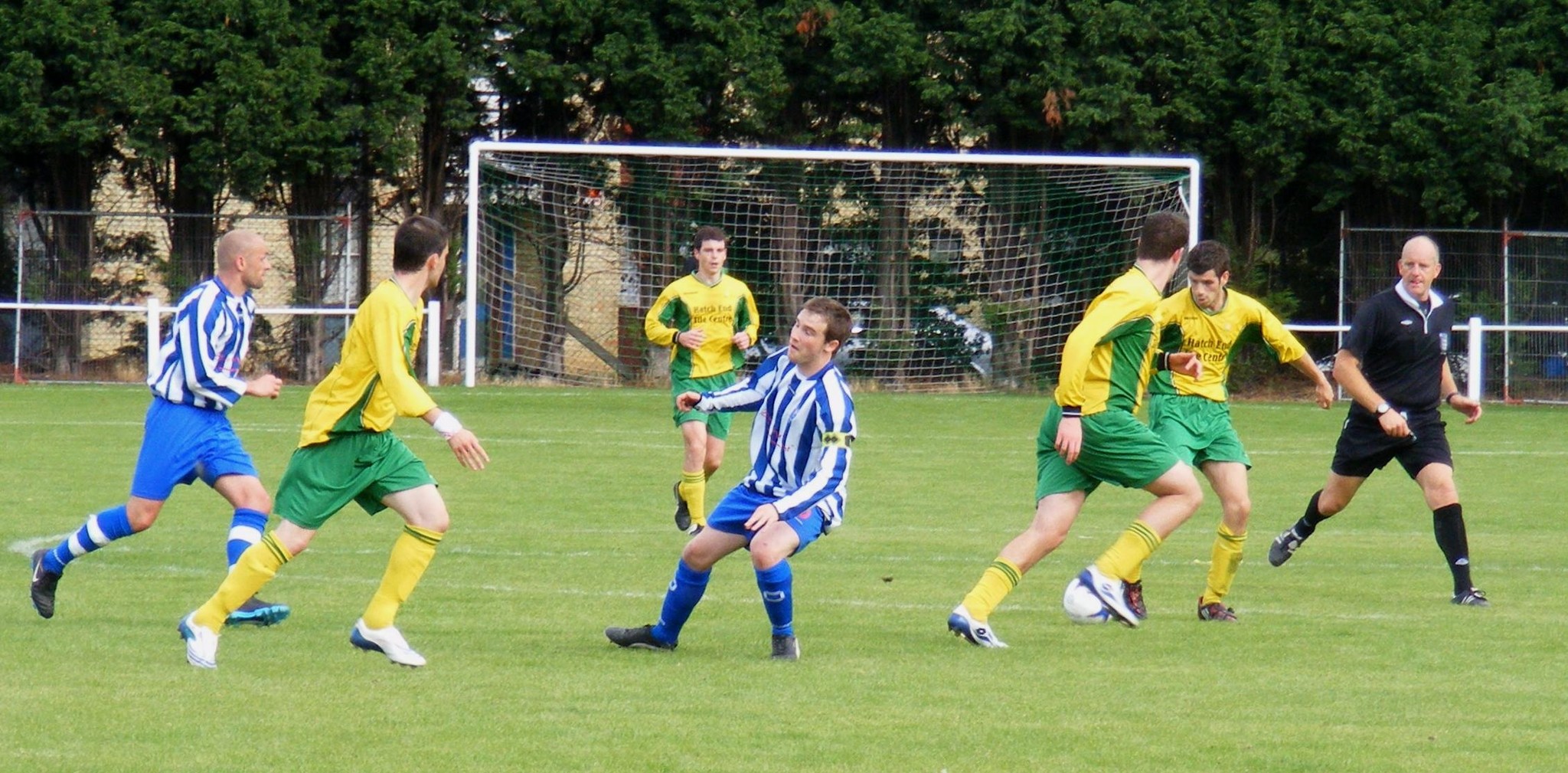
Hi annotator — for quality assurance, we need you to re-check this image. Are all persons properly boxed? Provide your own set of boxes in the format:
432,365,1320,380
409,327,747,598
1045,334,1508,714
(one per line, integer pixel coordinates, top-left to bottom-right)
644,225,760,535
947,210,1205,648
1268,235,1490,607
29,230,293,627
1120,241,1334,625
604,296,857,661
176,214,491,670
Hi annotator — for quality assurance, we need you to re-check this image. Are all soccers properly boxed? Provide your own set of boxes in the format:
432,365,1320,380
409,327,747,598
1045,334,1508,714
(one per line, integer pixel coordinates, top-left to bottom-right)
1064,578,1113,624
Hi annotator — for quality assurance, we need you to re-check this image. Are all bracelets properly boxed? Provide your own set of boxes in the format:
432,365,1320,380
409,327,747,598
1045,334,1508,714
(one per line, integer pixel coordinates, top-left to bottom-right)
1444,392,1461,404
430,410,463,442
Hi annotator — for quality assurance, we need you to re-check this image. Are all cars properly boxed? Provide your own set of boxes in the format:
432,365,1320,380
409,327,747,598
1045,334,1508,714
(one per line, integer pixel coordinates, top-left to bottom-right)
0,300,74,375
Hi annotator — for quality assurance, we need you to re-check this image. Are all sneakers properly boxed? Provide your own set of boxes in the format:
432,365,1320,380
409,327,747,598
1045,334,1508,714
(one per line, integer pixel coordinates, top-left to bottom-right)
688,523,706,536
31,548,63,619
770,629,798,660
672,481,693,530
947,604,1009,648
350,620,426,669
1077,566,1140,629
1451,586,1488,605
179,611,219,672
1123,579,1148,620
225,595,289,627
1198,596,1238,623
605,625,679,651
1267,524,1315,567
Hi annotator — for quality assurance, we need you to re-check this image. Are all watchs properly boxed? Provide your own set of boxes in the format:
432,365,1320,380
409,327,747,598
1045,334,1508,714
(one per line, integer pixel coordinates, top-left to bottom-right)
1373,402,1391,418
